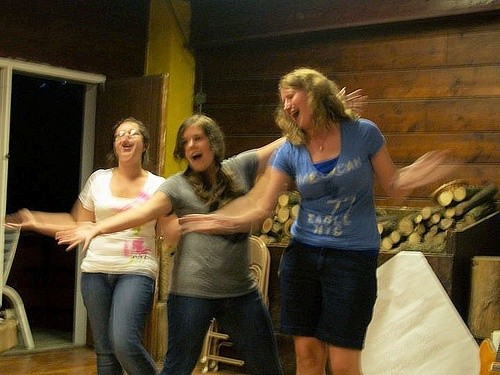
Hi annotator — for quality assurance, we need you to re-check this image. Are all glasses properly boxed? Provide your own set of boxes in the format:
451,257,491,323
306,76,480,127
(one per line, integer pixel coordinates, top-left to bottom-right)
114,129,144,138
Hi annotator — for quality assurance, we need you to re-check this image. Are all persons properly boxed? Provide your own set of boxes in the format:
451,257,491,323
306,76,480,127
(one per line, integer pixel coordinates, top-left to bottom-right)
171,68,457,375
55,87,369,375
2,117,167,375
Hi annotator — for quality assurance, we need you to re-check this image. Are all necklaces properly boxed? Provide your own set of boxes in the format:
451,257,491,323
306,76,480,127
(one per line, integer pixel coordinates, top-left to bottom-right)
306,130,330,151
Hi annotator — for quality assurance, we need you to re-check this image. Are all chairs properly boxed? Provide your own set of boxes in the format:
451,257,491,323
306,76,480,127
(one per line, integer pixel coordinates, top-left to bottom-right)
199,235,270,373
3,229,35,350
